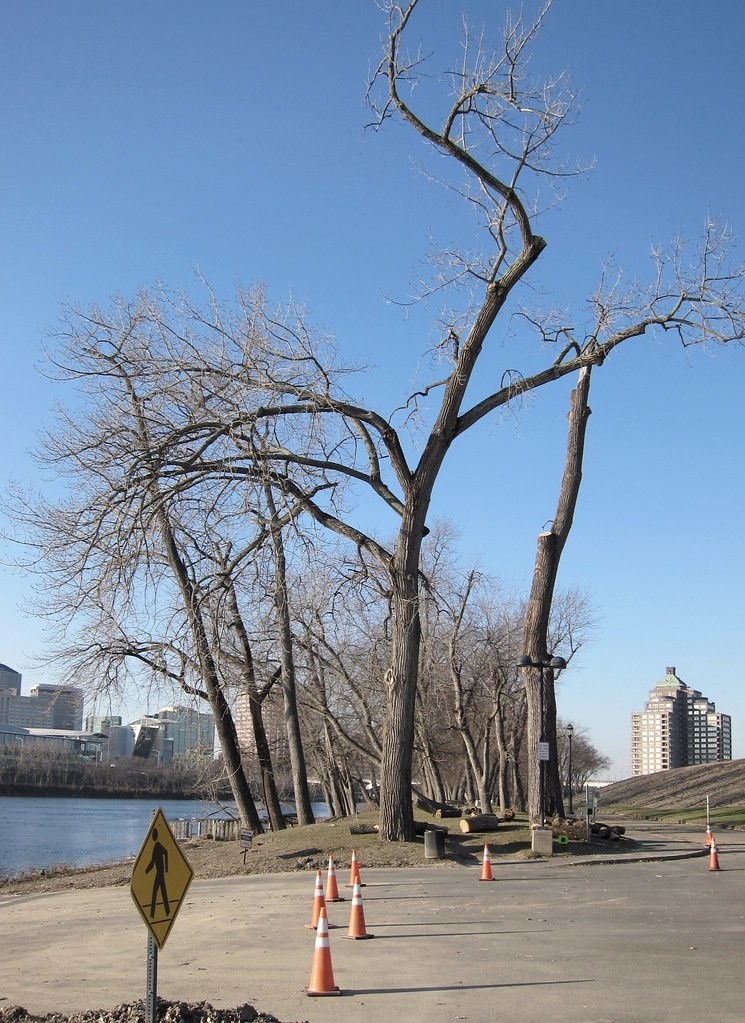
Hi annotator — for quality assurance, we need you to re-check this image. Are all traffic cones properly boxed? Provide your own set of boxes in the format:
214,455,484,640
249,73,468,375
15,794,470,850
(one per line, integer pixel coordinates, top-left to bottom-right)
479,843,496,881
705,824,713,847
305,907,341,996
345,848,366,887
342,875,374,940
304,870,335,930
324,854,346,903
708,835,722,871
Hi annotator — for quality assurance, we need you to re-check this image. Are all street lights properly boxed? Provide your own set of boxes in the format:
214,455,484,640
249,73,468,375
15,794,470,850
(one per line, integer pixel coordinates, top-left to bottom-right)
512,654,567,828
566,724,575,815
